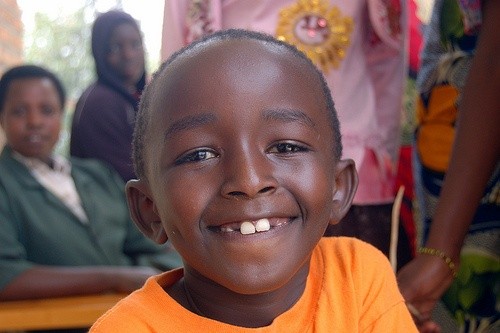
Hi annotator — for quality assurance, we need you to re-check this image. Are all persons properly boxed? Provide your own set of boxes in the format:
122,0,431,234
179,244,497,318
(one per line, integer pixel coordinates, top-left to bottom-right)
86,28,419,333
395,0,499,332
68,9,162,198
0,65,185,333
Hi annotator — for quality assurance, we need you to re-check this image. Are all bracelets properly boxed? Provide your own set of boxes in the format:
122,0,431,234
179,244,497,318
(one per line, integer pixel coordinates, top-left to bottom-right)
415,243,461,281
